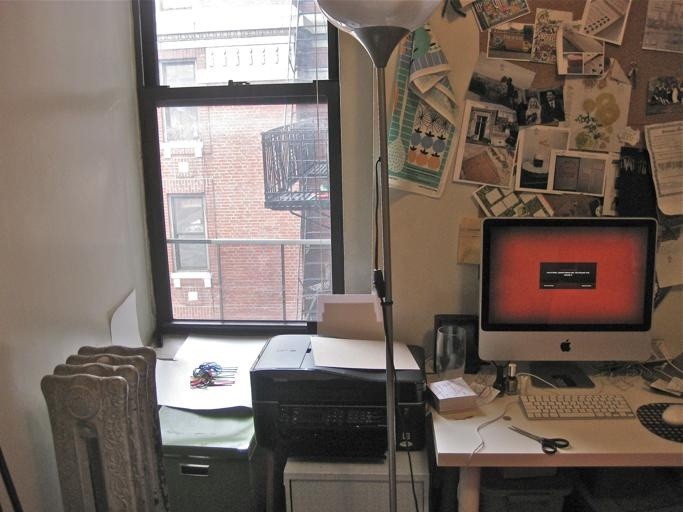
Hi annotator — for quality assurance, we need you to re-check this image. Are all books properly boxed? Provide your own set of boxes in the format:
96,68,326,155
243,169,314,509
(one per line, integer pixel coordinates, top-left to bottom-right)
425,376,478,413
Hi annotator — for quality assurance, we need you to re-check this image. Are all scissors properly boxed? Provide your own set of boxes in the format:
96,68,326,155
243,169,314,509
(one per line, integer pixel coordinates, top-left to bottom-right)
508,425,569,454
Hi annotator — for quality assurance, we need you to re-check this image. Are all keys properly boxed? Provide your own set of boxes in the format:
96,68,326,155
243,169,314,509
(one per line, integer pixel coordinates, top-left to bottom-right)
189,361,238,389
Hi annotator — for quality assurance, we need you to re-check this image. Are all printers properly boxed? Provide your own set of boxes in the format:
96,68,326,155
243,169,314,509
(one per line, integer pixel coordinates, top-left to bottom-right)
250,334,425,464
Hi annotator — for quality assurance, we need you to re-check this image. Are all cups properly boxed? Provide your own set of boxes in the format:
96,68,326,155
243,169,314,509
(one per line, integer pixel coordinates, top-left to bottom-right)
436,325,467,379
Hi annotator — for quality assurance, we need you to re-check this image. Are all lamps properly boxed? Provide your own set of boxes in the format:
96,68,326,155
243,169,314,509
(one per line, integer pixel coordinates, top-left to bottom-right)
314,1,442,511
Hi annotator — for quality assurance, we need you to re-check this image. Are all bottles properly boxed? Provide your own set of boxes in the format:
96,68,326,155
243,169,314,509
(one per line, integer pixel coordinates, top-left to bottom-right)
505,361,519,395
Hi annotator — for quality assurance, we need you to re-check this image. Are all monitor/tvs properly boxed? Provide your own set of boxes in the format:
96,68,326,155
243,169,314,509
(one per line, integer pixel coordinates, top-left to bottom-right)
478,216,658,388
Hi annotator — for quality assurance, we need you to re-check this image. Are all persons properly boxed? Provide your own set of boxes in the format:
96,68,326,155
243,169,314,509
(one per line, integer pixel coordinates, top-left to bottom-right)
541,91,565,123
525,98,542,124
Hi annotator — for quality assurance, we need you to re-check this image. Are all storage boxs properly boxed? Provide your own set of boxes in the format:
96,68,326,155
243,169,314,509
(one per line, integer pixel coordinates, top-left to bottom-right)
154,405,283,512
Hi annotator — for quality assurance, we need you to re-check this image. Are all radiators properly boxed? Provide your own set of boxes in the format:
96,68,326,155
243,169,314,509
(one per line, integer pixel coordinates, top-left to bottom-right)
40,346,170,511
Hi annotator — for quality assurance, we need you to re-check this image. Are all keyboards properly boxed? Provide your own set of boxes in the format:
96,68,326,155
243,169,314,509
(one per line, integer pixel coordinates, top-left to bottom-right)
519,392,637,420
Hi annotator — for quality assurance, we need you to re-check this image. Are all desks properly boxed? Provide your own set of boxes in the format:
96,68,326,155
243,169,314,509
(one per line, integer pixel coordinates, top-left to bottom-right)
424,350,682,511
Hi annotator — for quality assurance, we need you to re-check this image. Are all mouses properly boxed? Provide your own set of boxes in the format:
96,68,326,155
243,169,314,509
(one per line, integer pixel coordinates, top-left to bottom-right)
661,403,683,426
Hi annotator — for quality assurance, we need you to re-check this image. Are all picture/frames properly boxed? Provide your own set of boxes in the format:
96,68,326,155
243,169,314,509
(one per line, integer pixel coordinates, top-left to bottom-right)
431,312,480,374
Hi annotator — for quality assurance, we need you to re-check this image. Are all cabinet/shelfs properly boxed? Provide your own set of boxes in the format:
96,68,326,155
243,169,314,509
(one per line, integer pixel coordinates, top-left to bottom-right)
284,445,430,512
